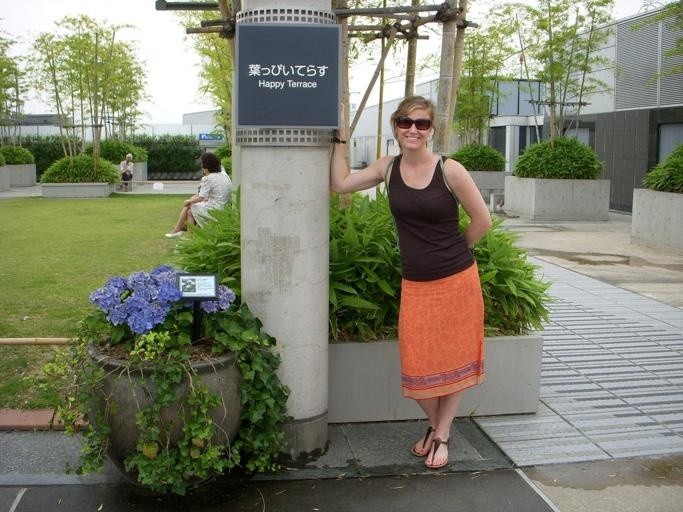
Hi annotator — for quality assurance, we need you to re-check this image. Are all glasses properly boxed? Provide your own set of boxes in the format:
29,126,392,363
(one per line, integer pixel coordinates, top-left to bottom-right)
394,116,433,131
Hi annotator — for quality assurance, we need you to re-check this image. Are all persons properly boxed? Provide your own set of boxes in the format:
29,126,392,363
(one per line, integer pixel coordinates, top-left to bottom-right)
120,153,134,190
329,96,492,469
163,151,232,239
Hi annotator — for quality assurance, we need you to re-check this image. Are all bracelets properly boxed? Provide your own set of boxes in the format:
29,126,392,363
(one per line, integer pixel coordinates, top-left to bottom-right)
334,138,347,144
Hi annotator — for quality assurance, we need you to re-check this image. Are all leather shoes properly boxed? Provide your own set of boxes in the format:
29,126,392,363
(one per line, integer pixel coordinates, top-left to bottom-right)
164,230,188,239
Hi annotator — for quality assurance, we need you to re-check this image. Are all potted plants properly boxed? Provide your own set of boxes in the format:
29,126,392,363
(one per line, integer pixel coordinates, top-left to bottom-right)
626,0,683,255
169,169,554,424
1,27,39,190
500,0,622,224
0,147,13,194
27,12,122,202
442,8,511,210
66,31,151,186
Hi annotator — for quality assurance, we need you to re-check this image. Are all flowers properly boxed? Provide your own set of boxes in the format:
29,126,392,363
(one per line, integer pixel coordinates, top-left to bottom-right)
35,263,296,500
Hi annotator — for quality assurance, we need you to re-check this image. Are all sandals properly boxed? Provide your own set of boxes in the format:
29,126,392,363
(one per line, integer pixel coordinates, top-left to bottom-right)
410,425,437,458
423,435,451,469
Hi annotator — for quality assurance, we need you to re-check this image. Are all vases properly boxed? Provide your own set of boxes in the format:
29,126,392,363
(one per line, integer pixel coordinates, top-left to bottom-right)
83,345,247,494
